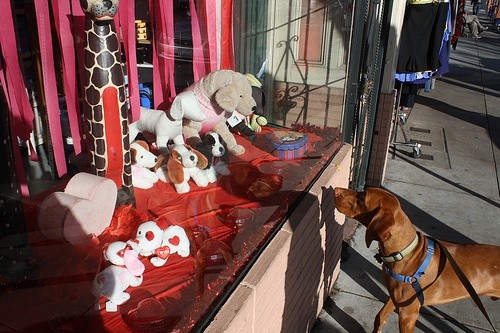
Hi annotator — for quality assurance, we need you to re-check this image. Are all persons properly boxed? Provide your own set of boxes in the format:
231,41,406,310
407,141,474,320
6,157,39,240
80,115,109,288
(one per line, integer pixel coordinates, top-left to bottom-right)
463,6,488,38
471,0,482,15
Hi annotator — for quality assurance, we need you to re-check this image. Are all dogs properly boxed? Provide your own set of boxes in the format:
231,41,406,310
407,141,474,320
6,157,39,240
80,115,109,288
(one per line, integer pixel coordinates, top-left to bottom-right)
330,187,499,333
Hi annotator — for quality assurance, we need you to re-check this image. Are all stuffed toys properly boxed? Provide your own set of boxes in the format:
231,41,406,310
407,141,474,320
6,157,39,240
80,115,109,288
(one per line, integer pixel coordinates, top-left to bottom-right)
180,69,257,156
154,144,208,194
91,239,145,312
191,132,230,183
226,109,268,135
130,140,159,190
128,91,207,147
135,213,190,267
